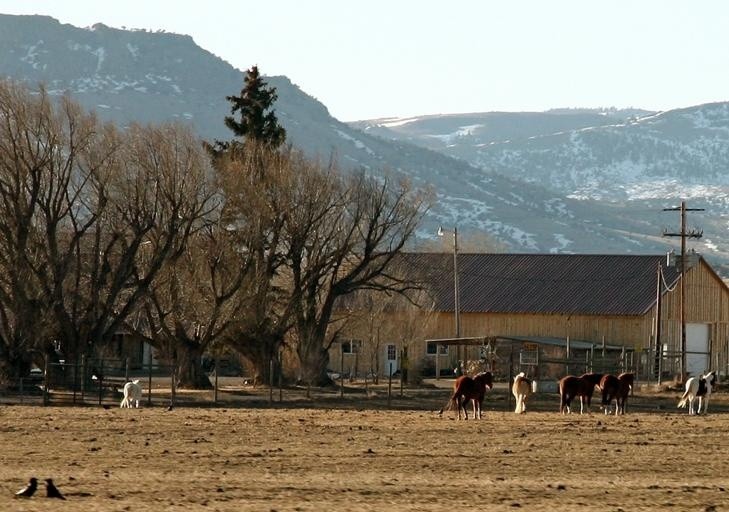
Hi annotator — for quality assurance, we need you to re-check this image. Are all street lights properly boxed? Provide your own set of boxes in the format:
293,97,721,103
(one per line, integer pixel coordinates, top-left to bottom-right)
437,226,460,368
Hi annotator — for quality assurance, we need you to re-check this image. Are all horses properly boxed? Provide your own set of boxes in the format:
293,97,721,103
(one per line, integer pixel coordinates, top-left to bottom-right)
558,373,604,416
510,371,534,415
677,370,719,416
119,379,142,408
445,369,494,420
599,371,635,416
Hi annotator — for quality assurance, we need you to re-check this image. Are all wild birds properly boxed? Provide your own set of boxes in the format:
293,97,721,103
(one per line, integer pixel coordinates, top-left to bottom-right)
42,478,67,501
14,476,40,499
